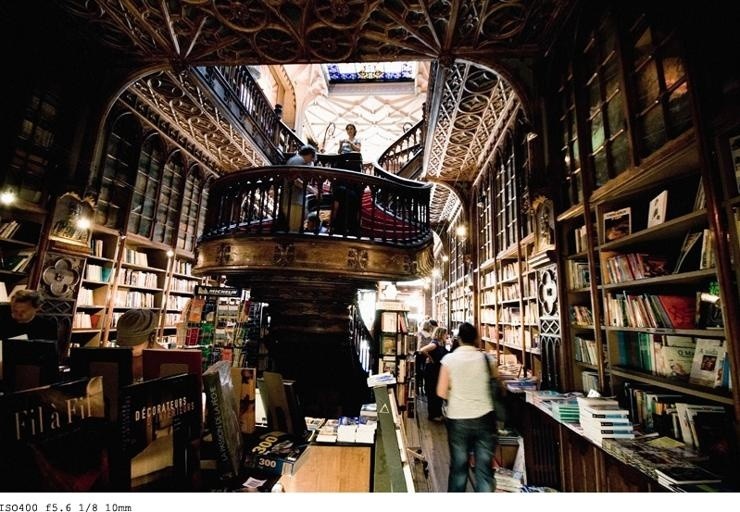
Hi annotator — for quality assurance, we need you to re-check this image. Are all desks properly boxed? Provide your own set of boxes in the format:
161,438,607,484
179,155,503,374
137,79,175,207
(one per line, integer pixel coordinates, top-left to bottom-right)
274,445,373,492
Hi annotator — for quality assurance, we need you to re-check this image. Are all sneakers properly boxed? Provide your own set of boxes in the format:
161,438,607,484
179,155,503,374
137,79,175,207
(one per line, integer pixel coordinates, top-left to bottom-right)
427,414,443,423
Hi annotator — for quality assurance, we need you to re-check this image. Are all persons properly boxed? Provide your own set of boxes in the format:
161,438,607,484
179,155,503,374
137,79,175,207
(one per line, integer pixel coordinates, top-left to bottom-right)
300,211,323,234
278,143,324,233
415,315,448,424
52,201,90,243
336,123,362,153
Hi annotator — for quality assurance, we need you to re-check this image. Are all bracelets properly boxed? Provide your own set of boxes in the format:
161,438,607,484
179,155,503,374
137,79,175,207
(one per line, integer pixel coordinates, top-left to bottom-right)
350,142,355,148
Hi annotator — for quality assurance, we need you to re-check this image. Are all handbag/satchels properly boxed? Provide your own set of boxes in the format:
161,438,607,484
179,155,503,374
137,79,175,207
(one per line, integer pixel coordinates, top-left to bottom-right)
485,354,511,421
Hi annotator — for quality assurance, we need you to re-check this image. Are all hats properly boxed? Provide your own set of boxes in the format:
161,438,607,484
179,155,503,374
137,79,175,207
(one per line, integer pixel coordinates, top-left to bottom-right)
115,307,158,345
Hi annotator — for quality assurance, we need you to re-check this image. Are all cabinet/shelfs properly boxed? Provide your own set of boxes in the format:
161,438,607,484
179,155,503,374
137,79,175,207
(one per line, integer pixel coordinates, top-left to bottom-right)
372,167,739,492
0,72,222,348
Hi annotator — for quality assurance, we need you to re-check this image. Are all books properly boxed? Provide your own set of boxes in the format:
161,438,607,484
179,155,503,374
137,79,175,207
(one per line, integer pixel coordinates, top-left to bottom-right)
301,371,398,447
434,160,739,492
1,358,316,492
0,217,217,350
378,310,417,419
